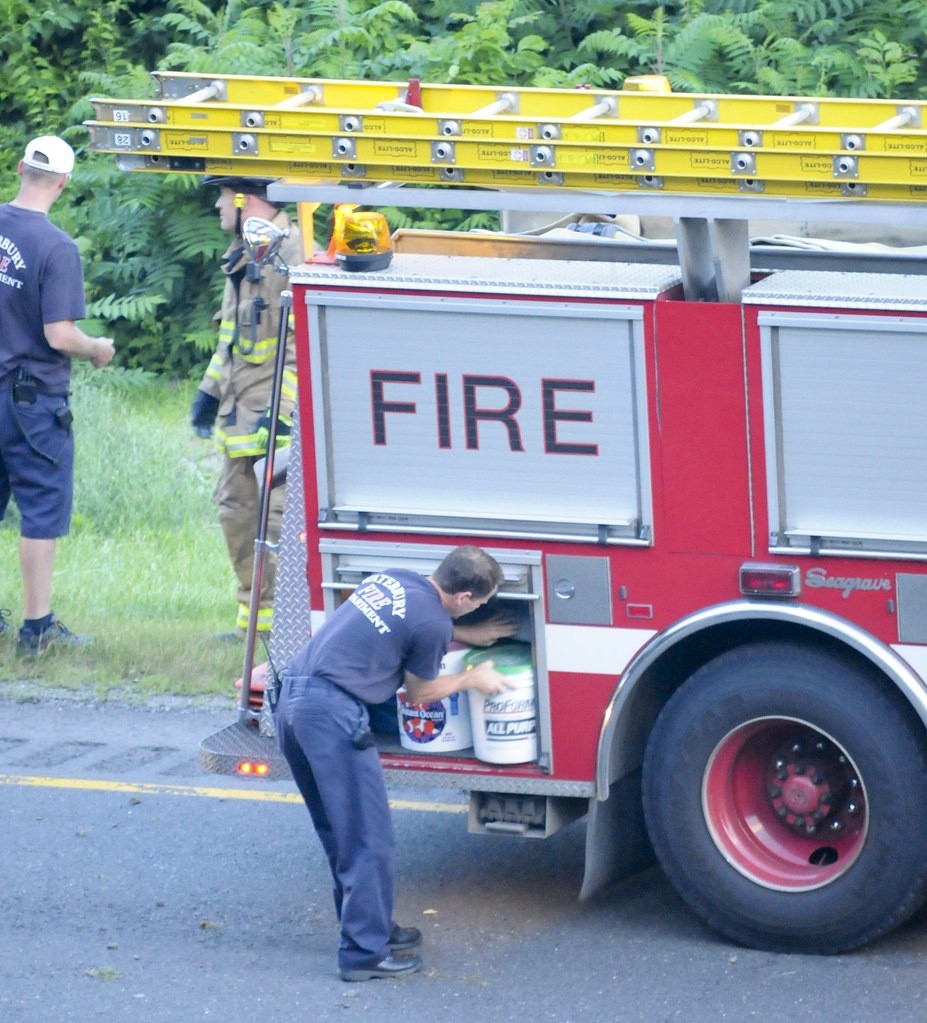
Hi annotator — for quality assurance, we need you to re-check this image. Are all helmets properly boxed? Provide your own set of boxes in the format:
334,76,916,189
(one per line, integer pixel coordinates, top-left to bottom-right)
201,174,289,208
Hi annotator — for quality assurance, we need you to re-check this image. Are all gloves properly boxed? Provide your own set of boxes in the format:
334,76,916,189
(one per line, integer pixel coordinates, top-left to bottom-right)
255,407,291,450
190,389,219,439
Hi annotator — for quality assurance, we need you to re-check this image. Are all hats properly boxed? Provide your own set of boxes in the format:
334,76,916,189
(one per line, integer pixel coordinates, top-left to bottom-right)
23,135,75,180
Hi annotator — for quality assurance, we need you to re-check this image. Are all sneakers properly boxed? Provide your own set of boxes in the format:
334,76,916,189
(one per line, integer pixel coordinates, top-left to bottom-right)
0,608,20,641
15,610,95,661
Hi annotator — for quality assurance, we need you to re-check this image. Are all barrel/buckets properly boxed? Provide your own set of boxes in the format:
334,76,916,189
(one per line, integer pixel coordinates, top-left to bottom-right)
462,642,539,765
395,640,473,754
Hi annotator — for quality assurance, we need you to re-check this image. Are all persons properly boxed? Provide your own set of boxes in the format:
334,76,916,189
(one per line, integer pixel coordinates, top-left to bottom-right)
273,545,519,982
1,133,116,658
190,172,333,641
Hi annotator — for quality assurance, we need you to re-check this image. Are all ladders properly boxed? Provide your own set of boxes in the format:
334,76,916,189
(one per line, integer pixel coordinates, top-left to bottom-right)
84,72,927,203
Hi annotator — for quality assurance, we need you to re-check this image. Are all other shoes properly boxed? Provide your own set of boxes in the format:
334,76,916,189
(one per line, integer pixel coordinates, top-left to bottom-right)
204,625,270,646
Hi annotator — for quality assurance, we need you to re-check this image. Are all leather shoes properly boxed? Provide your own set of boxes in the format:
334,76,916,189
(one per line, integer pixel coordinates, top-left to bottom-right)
385,921,422,950
339,951,421,981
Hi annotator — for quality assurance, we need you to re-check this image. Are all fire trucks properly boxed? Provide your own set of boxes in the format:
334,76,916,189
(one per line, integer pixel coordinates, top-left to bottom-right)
80,69,927,954
